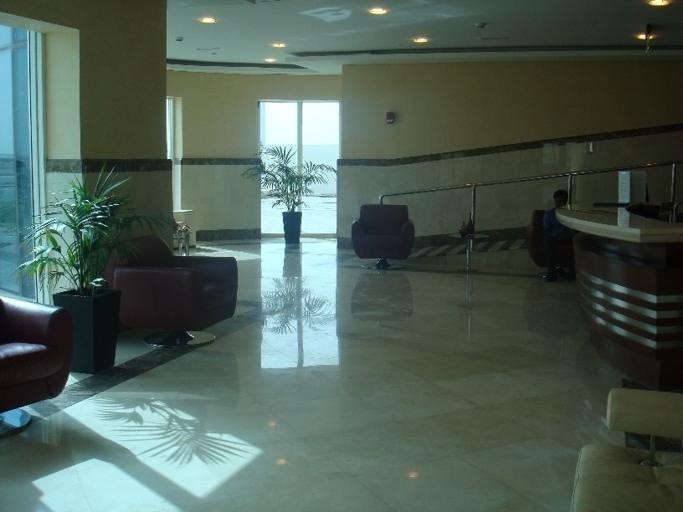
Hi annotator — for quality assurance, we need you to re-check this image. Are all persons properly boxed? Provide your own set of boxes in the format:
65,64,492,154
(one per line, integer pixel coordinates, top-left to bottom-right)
538,188,579,282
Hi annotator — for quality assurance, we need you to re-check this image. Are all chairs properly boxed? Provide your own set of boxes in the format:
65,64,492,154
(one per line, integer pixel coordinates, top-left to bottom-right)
529,208,570,282
572,386,683,511
0,297,73,436
110,234,238,348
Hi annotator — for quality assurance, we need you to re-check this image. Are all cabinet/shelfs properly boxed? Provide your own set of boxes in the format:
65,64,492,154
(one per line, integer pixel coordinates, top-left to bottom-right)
352,204,415,270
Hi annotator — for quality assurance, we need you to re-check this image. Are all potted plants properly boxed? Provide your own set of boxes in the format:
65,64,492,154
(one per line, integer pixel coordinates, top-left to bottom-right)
13,158,177,380
240,141,338,245
243,243,336,336
